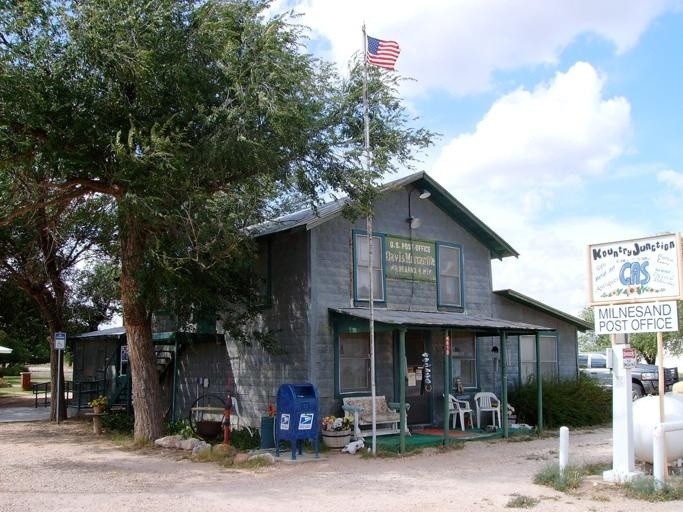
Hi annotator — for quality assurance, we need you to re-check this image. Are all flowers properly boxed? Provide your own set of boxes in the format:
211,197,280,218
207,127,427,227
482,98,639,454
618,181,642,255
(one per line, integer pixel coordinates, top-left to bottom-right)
86,392,107,408
321,409,357,430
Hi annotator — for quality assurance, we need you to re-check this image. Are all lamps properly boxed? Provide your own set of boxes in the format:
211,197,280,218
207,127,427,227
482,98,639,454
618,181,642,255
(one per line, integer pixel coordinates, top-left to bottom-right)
407,215,423,230
412,181,432,200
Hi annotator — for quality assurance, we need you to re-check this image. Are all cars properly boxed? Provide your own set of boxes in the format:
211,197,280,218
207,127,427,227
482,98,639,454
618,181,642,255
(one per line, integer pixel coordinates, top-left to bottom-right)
577,353,678,402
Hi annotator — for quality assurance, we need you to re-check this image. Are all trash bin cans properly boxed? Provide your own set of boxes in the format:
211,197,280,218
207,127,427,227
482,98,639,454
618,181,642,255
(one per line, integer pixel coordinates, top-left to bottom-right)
275,383,319,442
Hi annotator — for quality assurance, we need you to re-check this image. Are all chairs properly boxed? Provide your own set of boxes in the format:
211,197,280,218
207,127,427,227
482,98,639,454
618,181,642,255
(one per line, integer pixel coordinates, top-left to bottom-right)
442,390,503,431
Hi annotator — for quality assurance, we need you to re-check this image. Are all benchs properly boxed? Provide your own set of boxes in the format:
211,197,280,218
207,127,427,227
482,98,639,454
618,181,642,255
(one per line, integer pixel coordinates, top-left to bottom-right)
340,393,413,440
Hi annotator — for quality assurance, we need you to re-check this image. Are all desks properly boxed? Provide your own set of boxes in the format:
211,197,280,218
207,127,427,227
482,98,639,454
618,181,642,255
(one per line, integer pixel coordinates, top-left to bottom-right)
84,411,108,435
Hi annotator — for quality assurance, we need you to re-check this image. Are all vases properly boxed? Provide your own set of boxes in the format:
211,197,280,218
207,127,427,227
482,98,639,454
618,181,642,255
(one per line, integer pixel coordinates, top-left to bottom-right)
92,406,103,413
320,428,353,450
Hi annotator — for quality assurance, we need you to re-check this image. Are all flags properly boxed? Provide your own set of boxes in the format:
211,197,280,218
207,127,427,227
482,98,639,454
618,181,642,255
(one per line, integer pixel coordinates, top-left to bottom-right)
365,32,400,70
